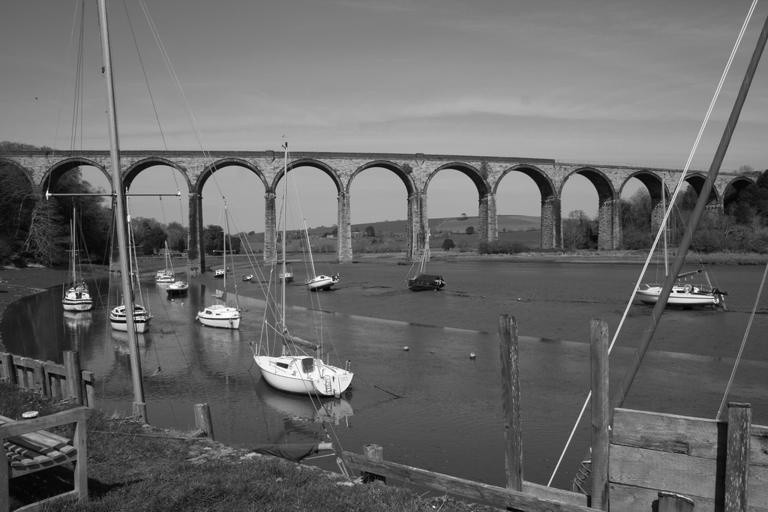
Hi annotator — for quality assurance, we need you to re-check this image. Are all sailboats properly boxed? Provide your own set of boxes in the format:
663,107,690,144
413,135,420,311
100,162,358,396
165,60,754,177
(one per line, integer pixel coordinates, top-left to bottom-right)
109,186,155,333
61,207,94,312
407,228,446,292
155,239,177,282
195,196,241,330
637,174,728,307
243,142,355,399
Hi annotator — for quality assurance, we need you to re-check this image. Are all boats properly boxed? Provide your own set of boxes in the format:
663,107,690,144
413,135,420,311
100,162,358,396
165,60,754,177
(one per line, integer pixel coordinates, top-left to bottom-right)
166,282,189,294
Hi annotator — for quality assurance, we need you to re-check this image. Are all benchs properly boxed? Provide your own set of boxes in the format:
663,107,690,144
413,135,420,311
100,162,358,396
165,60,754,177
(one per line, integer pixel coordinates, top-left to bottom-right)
0,406,92,512
